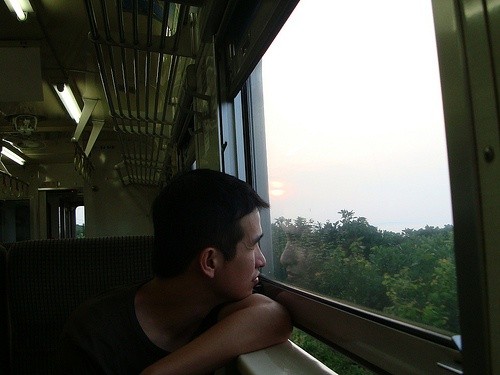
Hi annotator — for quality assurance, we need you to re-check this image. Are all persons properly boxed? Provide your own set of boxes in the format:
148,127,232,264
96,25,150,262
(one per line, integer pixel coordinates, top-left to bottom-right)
30,165,294,374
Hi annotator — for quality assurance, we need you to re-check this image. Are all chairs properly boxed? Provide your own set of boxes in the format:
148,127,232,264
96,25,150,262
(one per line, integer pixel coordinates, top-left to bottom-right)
0,236,161,374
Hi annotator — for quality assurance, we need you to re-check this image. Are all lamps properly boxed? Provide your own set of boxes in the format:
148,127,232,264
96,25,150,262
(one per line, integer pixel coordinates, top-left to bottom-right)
47,75,93,128
4,0,36,22
0,140,31,166
